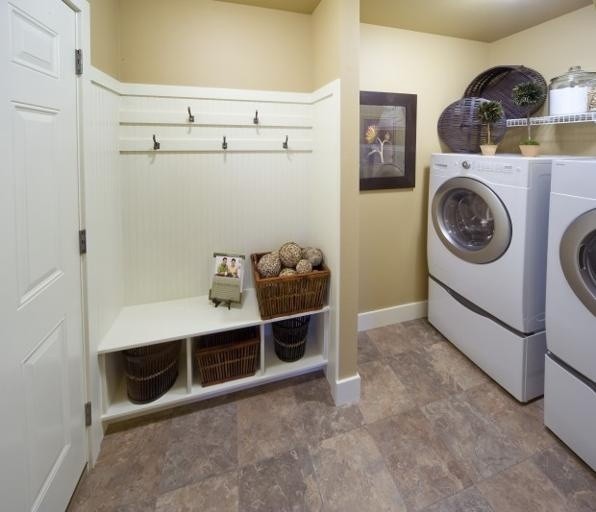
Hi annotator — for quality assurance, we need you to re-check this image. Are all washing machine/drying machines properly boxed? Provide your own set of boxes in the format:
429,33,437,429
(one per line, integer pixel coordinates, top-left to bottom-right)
426,154,549,406
544,157,596,473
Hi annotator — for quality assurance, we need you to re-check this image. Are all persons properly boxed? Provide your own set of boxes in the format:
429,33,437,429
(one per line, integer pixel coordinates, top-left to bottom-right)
228,258,241,278
216,256,229,277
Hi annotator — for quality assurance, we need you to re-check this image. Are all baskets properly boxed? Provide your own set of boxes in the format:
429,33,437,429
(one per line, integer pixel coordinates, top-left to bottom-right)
251,252,330,320
272,315,311,362
194,326,259,387
123,340,180,404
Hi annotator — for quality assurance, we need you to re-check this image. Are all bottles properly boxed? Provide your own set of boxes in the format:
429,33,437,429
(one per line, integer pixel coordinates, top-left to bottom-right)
548,62,596,115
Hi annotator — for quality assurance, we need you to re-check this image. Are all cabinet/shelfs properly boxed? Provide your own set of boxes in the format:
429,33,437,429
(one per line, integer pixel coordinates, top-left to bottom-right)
97,287,330,425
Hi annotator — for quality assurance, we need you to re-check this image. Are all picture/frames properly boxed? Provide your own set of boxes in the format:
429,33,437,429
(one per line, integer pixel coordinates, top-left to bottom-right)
360,91,416,189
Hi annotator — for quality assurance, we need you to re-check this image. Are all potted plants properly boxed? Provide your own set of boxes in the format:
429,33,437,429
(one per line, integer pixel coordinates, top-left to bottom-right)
475,101,504,156
510,80,547,157
